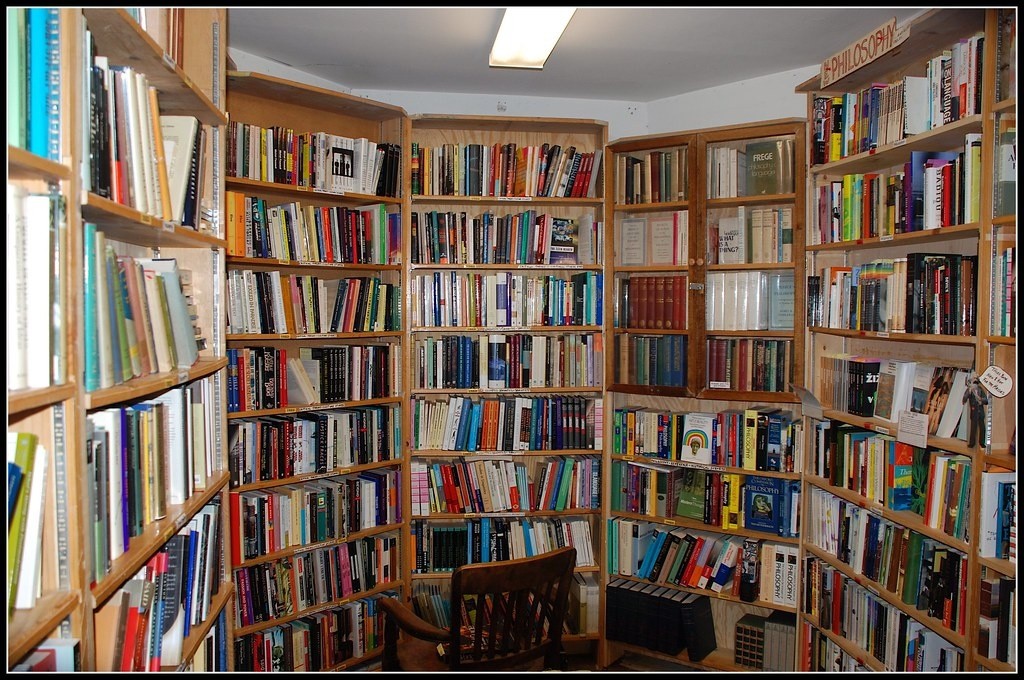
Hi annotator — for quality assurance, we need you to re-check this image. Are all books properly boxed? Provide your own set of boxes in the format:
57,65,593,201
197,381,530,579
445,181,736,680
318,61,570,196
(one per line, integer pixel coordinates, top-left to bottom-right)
8,8,1018,672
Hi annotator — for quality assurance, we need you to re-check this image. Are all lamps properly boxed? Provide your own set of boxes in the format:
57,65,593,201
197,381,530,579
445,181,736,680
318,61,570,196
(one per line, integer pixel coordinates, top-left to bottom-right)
488,7,578,70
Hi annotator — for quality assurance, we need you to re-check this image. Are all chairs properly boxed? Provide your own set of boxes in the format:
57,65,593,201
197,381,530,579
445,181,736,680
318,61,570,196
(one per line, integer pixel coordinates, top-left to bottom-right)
379,546,577,671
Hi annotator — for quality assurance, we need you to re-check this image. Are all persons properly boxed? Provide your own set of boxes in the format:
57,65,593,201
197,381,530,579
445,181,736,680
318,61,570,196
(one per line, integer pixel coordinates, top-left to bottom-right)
346,160,350,175
962,373,988,448
334,159,339,174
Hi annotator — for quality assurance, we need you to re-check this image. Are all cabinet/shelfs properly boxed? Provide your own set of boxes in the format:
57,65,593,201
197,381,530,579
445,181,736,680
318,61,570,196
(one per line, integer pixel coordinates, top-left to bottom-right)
8,7,1018,673
